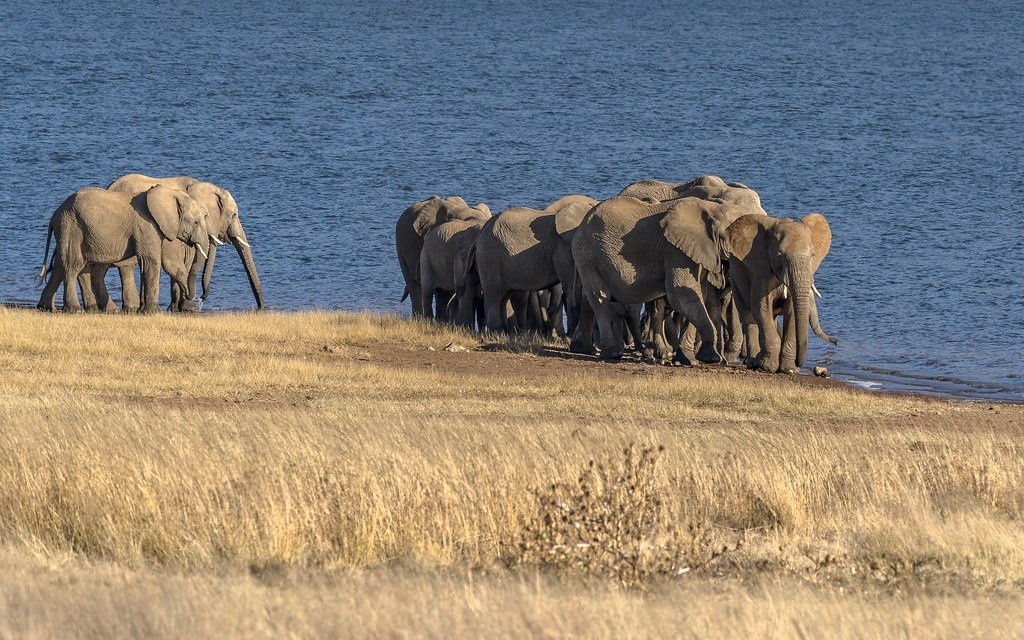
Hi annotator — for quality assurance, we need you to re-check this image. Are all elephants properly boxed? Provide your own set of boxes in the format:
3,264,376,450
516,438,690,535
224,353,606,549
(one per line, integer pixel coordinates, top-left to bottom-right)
395,174,831,375
33,174,265,313
